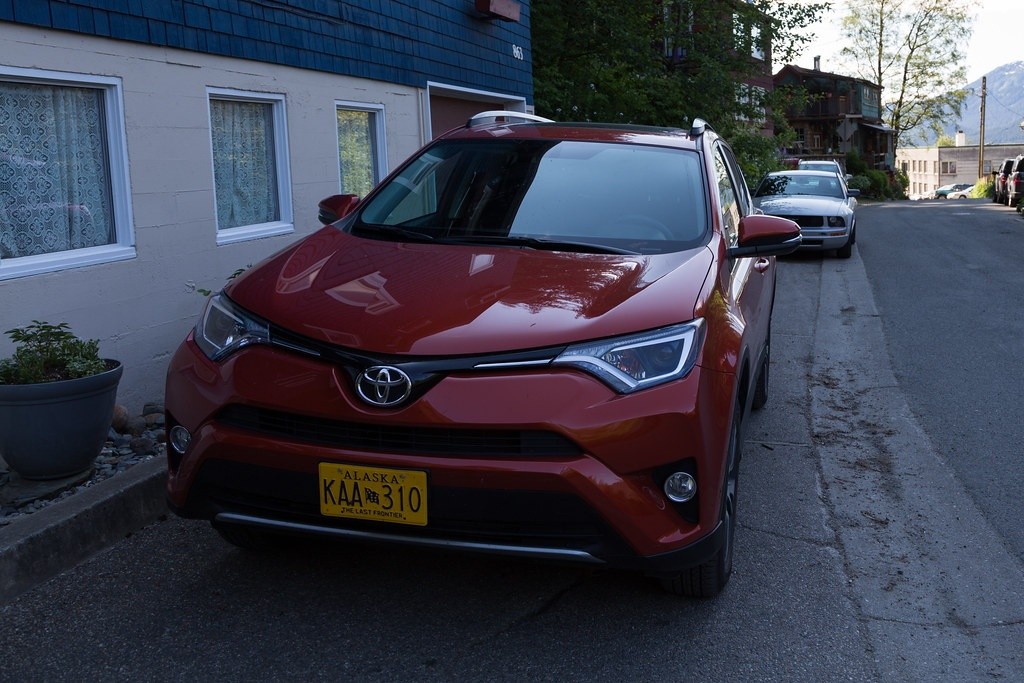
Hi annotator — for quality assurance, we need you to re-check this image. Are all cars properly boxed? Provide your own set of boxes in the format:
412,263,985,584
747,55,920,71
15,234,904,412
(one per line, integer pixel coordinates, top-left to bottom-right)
946,185,976,200
933,182,974,200
752,169,860,259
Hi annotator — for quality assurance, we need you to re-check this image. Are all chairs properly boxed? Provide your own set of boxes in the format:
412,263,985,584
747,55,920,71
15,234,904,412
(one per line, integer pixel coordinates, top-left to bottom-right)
448,172,526,236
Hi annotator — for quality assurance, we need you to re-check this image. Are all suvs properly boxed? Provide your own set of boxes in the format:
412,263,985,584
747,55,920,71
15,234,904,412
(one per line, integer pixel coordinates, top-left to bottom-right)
796,158,854,189
991,158,1015,203
165,110,806,601
1003,154,1024,207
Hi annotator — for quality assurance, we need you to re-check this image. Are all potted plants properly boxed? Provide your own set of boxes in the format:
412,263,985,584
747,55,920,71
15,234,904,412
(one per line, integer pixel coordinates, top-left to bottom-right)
0,320,124,481
